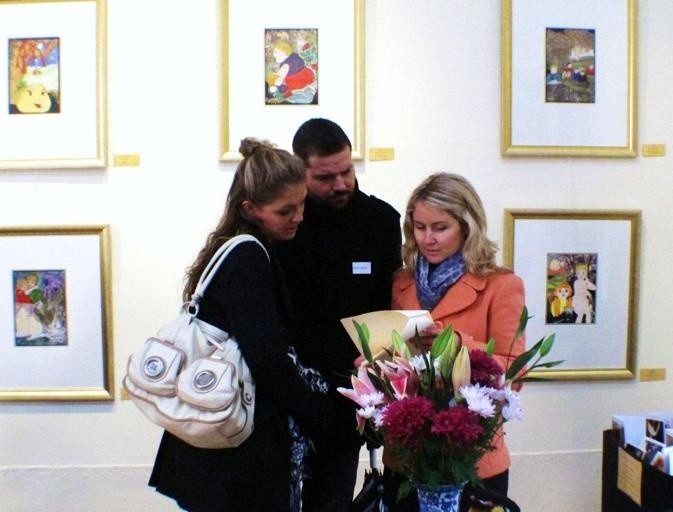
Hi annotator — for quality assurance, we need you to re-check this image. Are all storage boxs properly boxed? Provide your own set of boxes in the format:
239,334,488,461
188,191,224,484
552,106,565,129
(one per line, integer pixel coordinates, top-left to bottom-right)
600,427,673,512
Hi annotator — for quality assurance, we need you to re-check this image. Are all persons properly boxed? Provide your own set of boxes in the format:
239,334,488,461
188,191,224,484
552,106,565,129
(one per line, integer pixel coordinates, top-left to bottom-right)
146,136,336,512
353,171,526,503
15,274,54,339
268,42,317,105
573,263,596,324
550,283,572,317
283,117,402,512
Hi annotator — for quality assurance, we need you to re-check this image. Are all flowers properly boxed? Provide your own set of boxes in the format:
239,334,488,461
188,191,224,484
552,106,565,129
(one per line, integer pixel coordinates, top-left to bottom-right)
342,307,565,485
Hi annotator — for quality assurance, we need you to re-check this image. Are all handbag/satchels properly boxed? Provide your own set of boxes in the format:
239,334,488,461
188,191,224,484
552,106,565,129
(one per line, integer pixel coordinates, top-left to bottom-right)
122,233,272,450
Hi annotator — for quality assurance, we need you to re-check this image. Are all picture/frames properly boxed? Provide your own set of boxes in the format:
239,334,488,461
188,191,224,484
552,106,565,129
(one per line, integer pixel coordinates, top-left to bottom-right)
499,0,639,161
1,223,116,404
217,0,366,163
0,0,111,172
501,206,644,381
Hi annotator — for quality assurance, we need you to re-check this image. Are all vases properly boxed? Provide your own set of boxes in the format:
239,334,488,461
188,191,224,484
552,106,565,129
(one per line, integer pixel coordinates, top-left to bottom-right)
416,487,462,510
